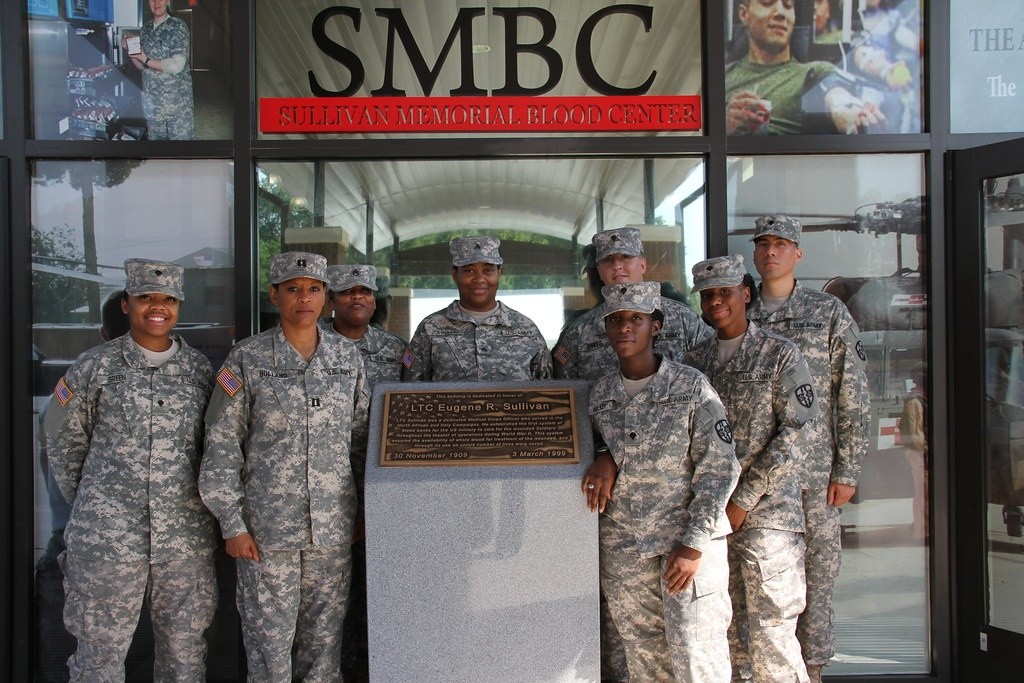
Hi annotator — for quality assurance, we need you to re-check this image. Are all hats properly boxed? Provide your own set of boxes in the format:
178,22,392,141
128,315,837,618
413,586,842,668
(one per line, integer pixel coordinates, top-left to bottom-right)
748,215,802,246
692,254,746,292
591,228,644,264
910,361,927,372
375,277,394,300
123,258,185,301
270,251,330,287
581,244,596,274
450,237,504,267
601,281,661,319
326,264,379,293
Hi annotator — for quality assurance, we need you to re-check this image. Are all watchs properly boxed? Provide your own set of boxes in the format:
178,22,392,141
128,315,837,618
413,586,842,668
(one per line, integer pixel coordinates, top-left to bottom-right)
596,446,608,455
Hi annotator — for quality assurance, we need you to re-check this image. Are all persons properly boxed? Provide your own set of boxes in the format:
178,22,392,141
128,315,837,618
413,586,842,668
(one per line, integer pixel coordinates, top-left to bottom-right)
402,236,554,380
745,215,872,683
726,0,919,137
551,228,714,683
99,290,132,342
683,254,820,683
370,275,408,345
126,0,193,140
581,281,742,683
43,259,219,683
321,266,409,683
901,362,929,546
581,244,605,308
197,251,372,683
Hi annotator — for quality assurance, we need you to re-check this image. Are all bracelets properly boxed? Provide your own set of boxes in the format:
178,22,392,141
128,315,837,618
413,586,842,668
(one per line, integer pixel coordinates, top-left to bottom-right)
144,59,151,69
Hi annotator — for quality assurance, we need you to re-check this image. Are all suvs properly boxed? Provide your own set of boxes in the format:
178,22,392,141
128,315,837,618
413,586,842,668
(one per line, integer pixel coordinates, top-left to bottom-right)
821,267,1024,536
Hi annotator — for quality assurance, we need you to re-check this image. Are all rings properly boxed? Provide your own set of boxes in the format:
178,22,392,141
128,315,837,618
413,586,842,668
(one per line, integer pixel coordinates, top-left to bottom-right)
588,484,594,488
